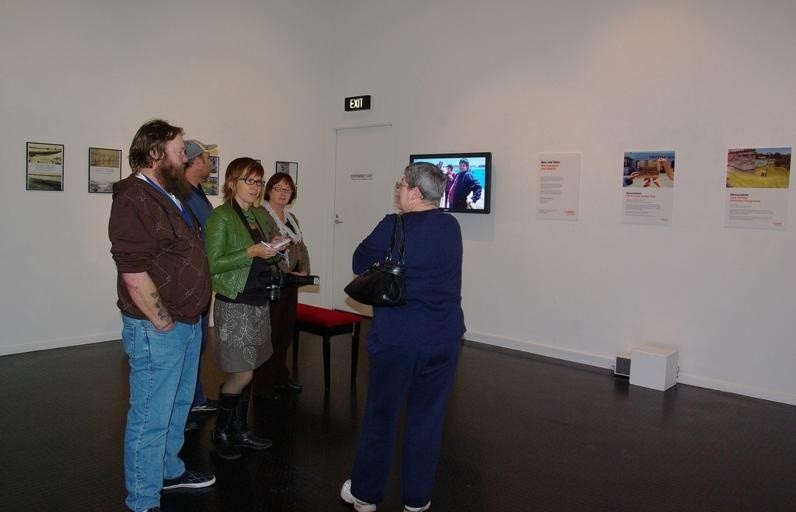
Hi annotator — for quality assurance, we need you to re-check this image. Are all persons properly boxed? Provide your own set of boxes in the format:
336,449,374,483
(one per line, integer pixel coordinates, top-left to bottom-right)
180,139,220,433
442,166,454,208
342,162,466,512
108,120,217,512
204,157,287,459
263,174,310,393
449,160,482,209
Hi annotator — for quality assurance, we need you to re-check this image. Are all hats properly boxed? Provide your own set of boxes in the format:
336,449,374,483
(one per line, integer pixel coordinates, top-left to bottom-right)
183,140,219,160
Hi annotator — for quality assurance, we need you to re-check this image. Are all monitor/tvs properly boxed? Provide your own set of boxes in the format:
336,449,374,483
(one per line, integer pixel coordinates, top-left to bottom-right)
409,151,492,215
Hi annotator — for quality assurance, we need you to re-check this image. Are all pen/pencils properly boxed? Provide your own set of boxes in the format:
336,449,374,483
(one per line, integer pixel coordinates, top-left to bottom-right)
260,241,284,256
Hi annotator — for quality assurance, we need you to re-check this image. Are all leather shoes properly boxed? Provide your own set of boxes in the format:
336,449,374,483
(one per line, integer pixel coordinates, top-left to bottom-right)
273,381,297,395
289,382,304,391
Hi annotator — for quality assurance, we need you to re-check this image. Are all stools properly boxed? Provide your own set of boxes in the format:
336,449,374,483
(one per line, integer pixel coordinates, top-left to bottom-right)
291,305,363,407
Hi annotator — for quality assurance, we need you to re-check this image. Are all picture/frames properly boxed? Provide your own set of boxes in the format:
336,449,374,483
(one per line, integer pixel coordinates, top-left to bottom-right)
200,155,220,197
275,161,299,189
24,142,66,191
86,148,122,194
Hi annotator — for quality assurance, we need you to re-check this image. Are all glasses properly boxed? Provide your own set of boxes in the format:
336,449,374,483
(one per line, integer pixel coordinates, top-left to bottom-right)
272,186,294,194
238,177,265,186
395,181,411,190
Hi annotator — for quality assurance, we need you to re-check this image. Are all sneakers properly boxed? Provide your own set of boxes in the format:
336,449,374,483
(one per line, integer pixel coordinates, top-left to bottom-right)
162,469,216,491
403,499,431,512
340,478,377,512
191,399,218,415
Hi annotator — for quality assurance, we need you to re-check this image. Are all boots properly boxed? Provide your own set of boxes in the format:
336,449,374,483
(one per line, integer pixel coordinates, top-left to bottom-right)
208,383,242,458
234,383,273,449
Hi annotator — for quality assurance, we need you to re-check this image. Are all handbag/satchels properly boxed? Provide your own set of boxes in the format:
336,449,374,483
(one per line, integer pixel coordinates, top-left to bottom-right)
343,213,409,308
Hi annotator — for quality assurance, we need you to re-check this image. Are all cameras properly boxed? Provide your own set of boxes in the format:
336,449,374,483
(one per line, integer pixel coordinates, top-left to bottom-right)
257,271,285,301
